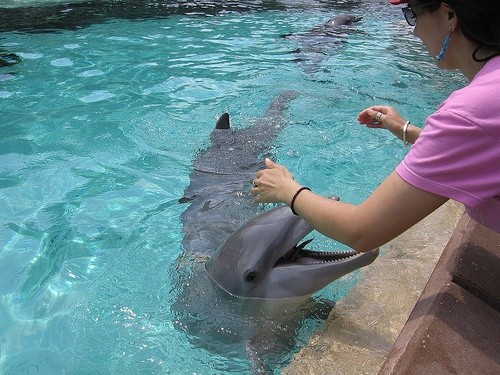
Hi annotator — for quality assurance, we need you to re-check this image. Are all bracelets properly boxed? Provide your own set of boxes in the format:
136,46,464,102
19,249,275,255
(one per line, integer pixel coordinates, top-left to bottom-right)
289,186,312,218
402,120,413,149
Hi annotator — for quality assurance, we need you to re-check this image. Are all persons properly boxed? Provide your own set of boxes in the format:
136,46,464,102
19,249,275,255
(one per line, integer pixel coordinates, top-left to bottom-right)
250,0,500,254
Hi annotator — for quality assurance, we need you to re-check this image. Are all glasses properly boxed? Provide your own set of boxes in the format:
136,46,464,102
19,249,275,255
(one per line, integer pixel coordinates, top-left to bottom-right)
402,2,441,26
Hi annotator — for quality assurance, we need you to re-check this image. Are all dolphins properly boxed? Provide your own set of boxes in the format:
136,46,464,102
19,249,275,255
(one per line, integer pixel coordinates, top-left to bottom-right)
277,14,371,76
168,90,379,374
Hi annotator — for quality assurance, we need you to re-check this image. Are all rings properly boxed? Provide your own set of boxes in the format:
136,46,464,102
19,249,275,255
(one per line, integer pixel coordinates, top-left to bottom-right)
252,178,258,188
376,112,383,121
